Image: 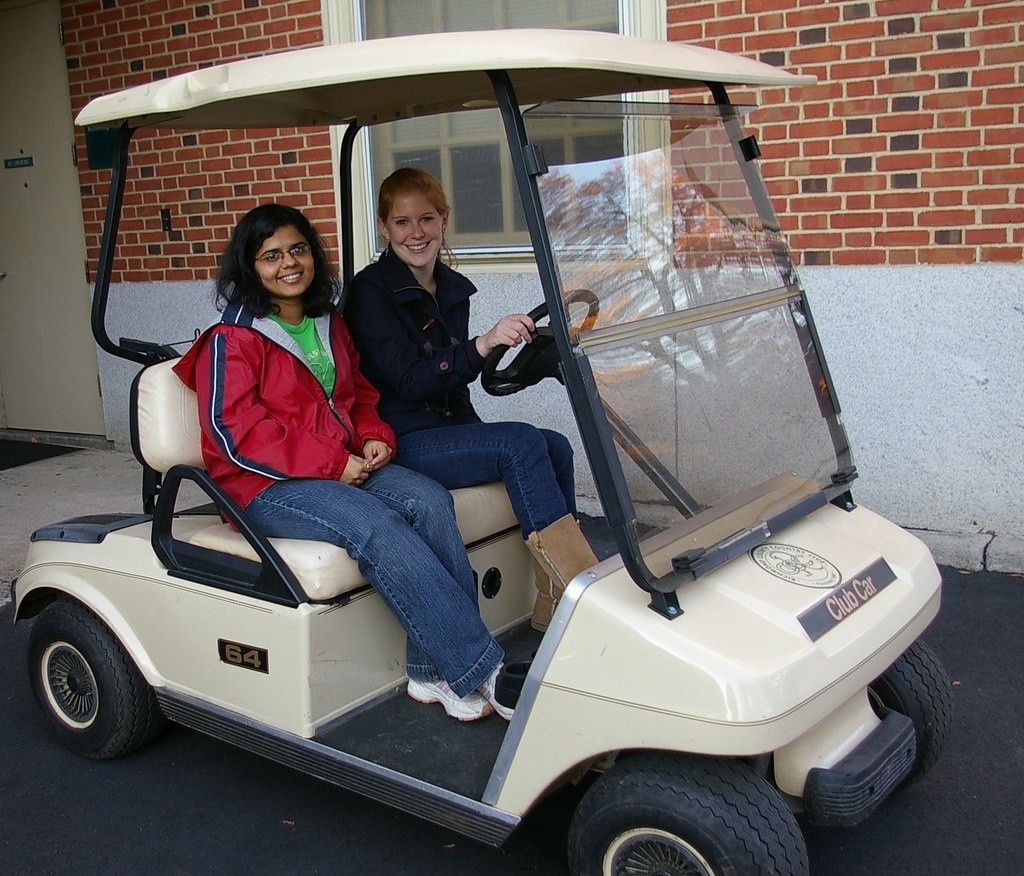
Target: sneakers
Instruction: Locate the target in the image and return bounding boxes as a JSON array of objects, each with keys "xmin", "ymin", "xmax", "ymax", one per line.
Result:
[
  {"xmin": 477, "ymin": 662, "xmax": 515, "ymax": 720},
  {"xmin": 407, "ymin": 678, "xmax": 494, "ymax": 721}
]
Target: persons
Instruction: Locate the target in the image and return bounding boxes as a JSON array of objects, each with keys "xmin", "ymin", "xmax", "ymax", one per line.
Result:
[
  {"xmin": 337, "ymin": 168, "xmax": 601, "ymax": 633},
  {"xmin": 172, "ymin": 204, "xmax": 524, "ymax": 722}
]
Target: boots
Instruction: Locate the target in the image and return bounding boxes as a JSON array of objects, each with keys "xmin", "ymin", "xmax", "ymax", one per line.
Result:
[
  {"xmin": 524, "ymin": 513, "xmax": 599, "ymax": 590},
  {"xmin": 530, "ymin": 553, "xmax": 563, "ymax": 632}
]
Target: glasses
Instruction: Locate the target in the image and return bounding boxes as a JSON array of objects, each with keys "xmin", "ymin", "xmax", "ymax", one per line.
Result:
[{"xmin": 254, "ymin": 244, "xmax": 312, "ymax": 265}]
